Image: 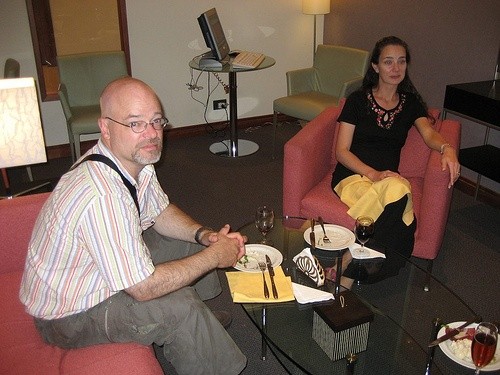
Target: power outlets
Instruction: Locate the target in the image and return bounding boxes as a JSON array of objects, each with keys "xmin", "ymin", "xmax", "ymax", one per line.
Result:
[{"xmin": 213, "ymin": 99, "xmax": 226, "ymax": 109}]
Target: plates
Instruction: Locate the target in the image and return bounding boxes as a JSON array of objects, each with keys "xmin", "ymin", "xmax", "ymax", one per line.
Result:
[
  {"xmin": 303, "ymin": 224, "xmax": 356, "ymax": 251},
  {"xmin": 233, "ymin": 244, "xmax": 283, "ymax": 272},
  {"xmin": 437, "ymin": 321, "xmax": 500, "ymax": 371}
]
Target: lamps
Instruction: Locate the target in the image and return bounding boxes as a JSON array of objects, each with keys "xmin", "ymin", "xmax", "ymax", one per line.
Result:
[
  {"xmin": 303, "ymin": 0, "xmax": 330, "ymax": 55},
  {"xmin": 0, "ymin": 78, "xmax": 49, "ymax": 182}
]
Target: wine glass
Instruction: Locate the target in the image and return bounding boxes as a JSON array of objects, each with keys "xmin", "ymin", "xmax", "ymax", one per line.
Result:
[
  {"xmin": 353, "ymin": 216, "xmax": 374, "ymax": 257},
  {"xmin": 471, "ymin": 322, "xmax": 498, "ymax": 375},
  {"xmin": 255, "ymin": 206, "xmax": 274, "ymax": 245}
]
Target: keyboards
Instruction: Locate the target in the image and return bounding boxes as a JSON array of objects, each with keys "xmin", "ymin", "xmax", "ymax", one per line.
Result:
[{"xmin": 233, "ymin": 52, "xmax": 266, "ymax": 69}]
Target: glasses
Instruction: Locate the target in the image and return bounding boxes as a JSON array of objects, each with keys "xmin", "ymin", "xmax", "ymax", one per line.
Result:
[{"xmin": 103, "ymin": 116, "xmax": 168, "ymax": 133}]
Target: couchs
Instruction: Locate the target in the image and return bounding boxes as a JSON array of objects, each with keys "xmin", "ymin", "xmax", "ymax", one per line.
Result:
[{"xmin": 0, "ymin": 193, "xmax": 164, "ymax": 375}]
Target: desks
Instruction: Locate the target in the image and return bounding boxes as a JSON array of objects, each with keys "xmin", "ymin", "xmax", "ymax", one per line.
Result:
[{"xmin": 190, "ymin": 51, "xmax": 276, "ymax": 157}]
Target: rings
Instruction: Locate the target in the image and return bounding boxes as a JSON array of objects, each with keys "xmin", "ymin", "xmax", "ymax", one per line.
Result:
[{"xmin": 457, "ymin": 172, "xmax": 461, "ymax": 173}]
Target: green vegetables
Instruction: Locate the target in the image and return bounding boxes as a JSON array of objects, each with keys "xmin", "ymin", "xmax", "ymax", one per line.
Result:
[{"xmin": 239, "ymin": 255, "xmax": 248, "ymax": 268}]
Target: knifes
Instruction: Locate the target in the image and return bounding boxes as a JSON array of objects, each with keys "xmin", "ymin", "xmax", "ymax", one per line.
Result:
[
  {"xmin": 309, "ymin": 217, "xmax": 315, "ymax": 250},
  {"xmin": 265, "ymin": 254, "xmax": 278, "ymax": 299},
  {"xmin": 426, "ymin": 319, "xmax": 478, "ymax": 347}
]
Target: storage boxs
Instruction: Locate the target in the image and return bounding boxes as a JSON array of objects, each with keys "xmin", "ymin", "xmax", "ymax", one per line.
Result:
[{"xmin": 311, "ymin": 290, "xmax": 374, "ymax": 362}]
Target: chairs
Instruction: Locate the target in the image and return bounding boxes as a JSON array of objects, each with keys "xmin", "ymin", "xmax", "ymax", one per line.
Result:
[
  {"xmin": 283, "ymin": 98, "xmax": 461, "ymax": 292},
  {"xmin": 273, "ymin": 45, "xmax": 370, "ymax": 160},
  {"xmin": 55, "ymin": 51, "xmax": 130, "ymax": 164}
]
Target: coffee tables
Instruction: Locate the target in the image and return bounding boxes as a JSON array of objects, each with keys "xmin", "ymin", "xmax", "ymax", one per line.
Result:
[{"xmin": 226, "ymin": 216, "xmax": 500, "ymax": 375}]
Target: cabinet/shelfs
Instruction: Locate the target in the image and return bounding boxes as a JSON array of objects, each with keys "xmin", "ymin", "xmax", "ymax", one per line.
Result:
[{"xmin": 443, "ymin": 78, "xmax": 500, "ymax": 200}]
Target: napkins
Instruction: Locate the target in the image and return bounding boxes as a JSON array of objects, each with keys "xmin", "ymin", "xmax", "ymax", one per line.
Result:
[
  {"xmin": 334, "ymin": 173, "xmax": 414, "ymax": 226},
  {"xmin": 349, "ymin": 243, "xmax": 386, "ymax": 260},
  {"xmin": 224, "ymin": 266, "xmax": 295, "ymax": 303},
  {"xmin": 292, "ymin": 282, "xmax": 335, "ymax": 305}
]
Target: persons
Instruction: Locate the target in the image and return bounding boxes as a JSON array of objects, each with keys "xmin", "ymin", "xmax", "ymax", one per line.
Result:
[
  {"xmin": 323, "ymin": 37, "xmax": 461, "ymax": 293},
  {"xmin": 18, "ymin": 77, "xmax": 249, "ymax": 374}
]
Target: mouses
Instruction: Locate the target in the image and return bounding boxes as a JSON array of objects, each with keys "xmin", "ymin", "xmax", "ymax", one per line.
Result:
[{"xmin": 228, "ymin": 49, "xmax": 241, "ymax": 55}]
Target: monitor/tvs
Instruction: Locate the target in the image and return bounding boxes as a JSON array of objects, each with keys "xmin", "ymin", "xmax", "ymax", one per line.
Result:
[{"xmin": 197, "ymin": 7, "xmax": 231, "ymax": 68}]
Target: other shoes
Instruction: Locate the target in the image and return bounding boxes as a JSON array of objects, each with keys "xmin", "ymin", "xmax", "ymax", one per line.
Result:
[{"xmin": 212, "ymin": 311, "xmax": 231, "ymax": 327}]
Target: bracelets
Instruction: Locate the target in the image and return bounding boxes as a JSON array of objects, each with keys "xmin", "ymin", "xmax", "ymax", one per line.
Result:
[
  {"xmin": 195, "ymin": 226, "xmax": 213, "ymax": 245},
  {"xmin": 440, "ymin": 143, "xmax": 451, "ymax": 154}
]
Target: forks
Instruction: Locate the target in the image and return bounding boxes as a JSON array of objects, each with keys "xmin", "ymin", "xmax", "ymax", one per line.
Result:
[
  {"xmin": 318, "ymin": 216, "xmax": 331, "ymax": 243},
  {"xmin": 258, "ymin": 261, "xmax": 270, "ymax": 299}
]
